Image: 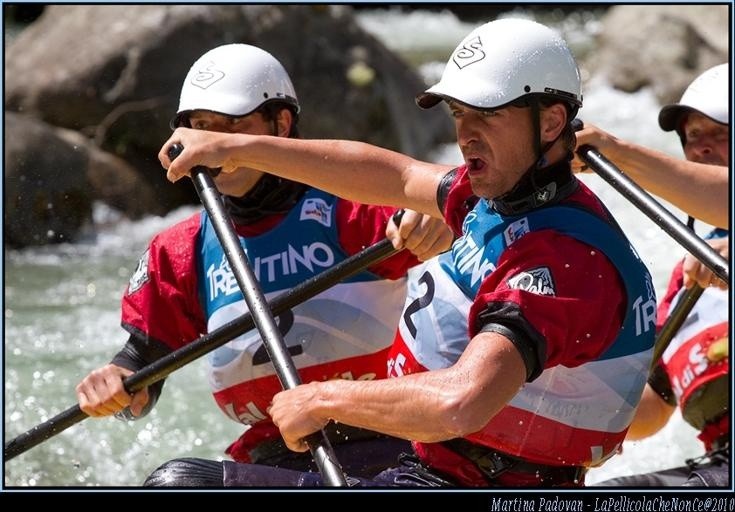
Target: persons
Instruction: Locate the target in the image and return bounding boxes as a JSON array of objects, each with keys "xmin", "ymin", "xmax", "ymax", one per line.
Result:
[
  {"xmin": 74, "ymin": 43, "xmax": 459, "ymax": 490},
  {"xmin": 592, "ymin": 60, "xmax": 728, "ymax": 487},
  {"xmin": 158, "ymin": 15, "xmax": 656, "ymax": 488},
  {"xmin": 568, "ymin": 117, "xmax": 729, "ymax": 230}
]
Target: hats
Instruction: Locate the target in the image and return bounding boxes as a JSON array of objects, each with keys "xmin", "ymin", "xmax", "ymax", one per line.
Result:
[
  {"xmin": 415, "ymin": 17, "xmax": 584, "ymax": 113},
  {"xmin": 174, "ymin": 42, "xmax": 303, "ymax": 118},
  {"xmin": 657, "ymin": 63, "xmax": 730, "ymax": 131}
]
